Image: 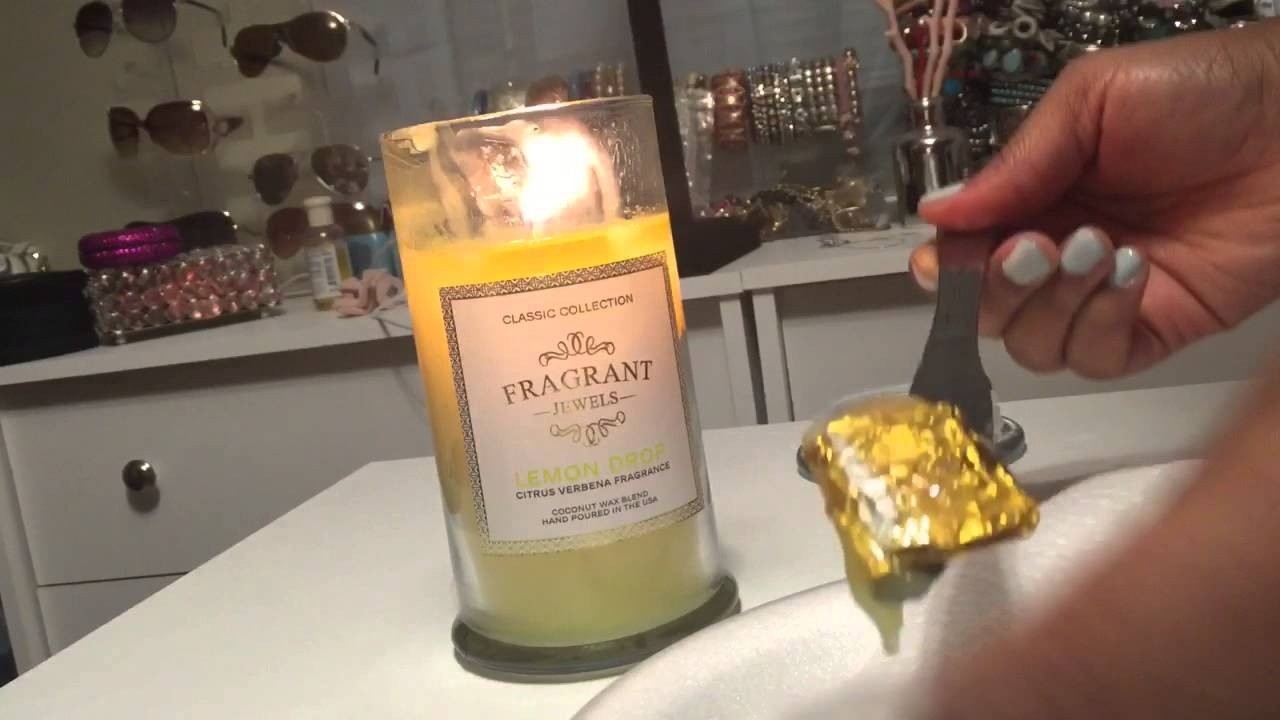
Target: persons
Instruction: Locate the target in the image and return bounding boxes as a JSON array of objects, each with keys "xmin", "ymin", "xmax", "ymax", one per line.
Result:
[{"xmin": 905, "ymin": 14, "xmax": 1280, "ymax": 720}]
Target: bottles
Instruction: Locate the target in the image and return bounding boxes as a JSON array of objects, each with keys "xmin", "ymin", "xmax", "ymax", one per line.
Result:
[{"xmin": 302, "ymin": 195, "xmax": 355, "ymax": 312}]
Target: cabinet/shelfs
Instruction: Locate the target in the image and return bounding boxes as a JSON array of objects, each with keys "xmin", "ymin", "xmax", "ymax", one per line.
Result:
[{"xmin": 0, "ymin": 213, "xmax": 1280, "ymax": 680}]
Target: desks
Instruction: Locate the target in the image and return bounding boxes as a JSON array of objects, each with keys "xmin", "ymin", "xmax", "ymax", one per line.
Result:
[{"xmin": 0, "ymin": 381, "xmax": 1250, "ymax": 720}]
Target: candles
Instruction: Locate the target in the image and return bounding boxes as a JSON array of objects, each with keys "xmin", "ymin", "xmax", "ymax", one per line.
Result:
[{"xmin": 403, "ymin": 131, "xmax": 724, "ymax": 646}]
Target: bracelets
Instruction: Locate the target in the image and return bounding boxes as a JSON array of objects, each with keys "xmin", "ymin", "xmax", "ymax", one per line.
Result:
[
  {"xmin": 672, "ymin": 48, "xmax": 862, "ymax": 159},
  {"xmin": 473, "ymin": 63, "xmax": 627, "ymax": 115}
]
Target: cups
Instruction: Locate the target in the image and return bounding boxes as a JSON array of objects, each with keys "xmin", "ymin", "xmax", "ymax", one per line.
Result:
[{"xmin": 379, "ymin": 93, "xmax": 744, "ymax": 684}]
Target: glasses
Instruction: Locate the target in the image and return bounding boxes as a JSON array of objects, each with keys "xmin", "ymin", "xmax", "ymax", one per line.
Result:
[
  {"xmin": 230, "ymin": 12, "xmax": 380, "ymax": 76},
  {"xmin": 71, "ymin": 0, "xmax": 228, "ymax": 59},
  {"xmin": 265, "ymin": 200, "xmax": 382, "ymax": 260},
  {"xmin": 109, "ymin": 101, "xmax": 241, "ymax": 156},
  {"xmin": 248, "ymin": 144, "xmax": 382, "ymax": 206}
]
